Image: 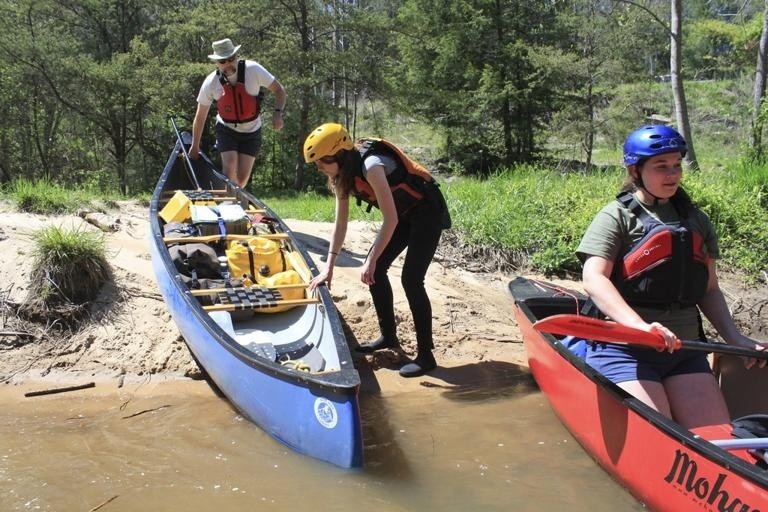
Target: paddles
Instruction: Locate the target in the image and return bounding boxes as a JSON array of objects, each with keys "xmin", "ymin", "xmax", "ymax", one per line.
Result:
[
  {"xmin": 166, "ymin": 114, "xmax": 217, "ymax": 206},
  {"xmin": 533, "ymin": 314, "xmax": 768, "ymax": 362}
]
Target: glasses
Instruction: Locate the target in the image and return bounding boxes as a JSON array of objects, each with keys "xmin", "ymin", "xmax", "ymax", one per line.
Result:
[{"xmin": 217, "ymin": 54, "xmax": 237, "ymax": 64}]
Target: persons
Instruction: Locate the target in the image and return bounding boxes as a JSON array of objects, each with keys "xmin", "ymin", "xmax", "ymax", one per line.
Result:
[
  {"xmin": 302, "ymin": 123, "xmax": 451, "ymax": 376},
  {"xmin": 187, "ymin": 38, "xmax": 286, "ymax": 186},
  {"xmin": 574, "ymin": 124, "xmax": 767, "ymax": 430}
]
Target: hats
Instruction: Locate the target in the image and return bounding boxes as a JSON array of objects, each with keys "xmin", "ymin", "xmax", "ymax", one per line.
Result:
[{"xmin": 208, "ymin": 38, "xmax": 241, "ymax": 60}]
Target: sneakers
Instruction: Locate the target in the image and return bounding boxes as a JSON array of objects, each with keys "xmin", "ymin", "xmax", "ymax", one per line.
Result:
[
  {"xmin": 355, "ymin": 334, "xmax": 399, "ymax": 353},
  {"xmin": 399, "ymin": 351, "xmax": 437, "ymax": 377}
]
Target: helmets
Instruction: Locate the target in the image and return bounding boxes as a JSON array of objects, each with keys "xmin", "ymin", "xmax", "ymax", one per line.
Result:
[
  {"xmin": 623, "ymin": 123, "xmax": 689, "ymax": 167},
  {"xmin": 302, "ymin": 123, "xmax": 354, "ymax": 164}
]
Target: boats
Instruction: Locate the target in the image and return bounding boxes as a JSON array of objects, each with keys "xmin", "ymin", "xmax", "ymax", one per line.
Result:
[
  {"xmin": 506, "ymin": 276, "xmax": 768, "ymax": 512},
  {"xmin": 149, "ymin": 130, "xmax": 365, "ymax": 470}
]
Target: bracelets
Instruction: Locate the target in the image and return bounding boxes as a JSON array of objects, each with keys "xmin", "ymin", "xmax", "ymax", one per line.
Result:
[
  {"xmin": 274, "ymin": 108, "xmax": 283, "ymax": 113},
  {"xmin": 328, "ymin": 251, "xmax": 338, "ymax": 255}
]
